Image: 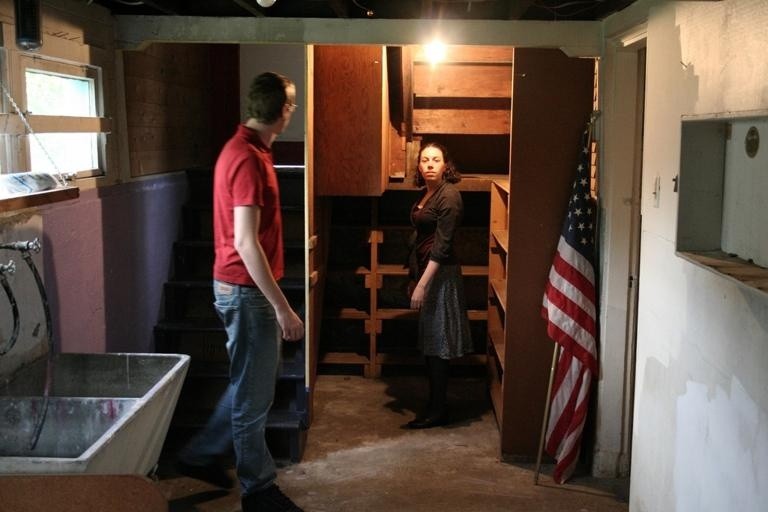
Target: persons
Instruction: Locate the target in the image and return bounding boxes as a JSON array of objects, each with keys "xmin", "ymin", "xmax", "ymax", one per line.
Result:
[
  {"xmin": 403, "ymin": 141, "xmax": 484, "ymax": 430},
  {"xmin": 175, "ymin": 70, "xmax": 307, "ymax": 512}
]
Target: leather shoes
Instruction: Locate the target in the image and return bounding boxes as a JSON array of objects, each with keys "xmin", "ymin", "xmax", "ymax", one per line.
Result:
[{"xmin": 407, "ymin": 403, "xmax": 452, "ymax": 428}]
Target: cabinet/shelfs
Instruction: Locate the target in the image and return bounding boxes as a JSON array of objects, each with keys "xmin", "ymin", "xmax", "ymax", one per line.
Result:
[
  {"xmin": 319, "ymin": 172, "xmax": 490, "ymax": 380},
  {"xmin": 487, "ymin": 173, "xmax": 581, "ymax": 462}
]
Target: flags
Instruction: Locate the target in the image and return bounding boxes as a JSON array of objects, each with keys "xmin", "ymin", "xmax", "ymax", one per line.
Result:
[{"xmin": 540, "ymin": 132, "xmax": 601, "ymax": 485}]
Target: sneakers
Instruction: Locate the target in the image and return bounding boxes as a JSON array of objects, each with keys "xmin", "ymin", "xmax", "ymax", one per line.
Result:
[
  {"xmin": 240, "ymin": 483, "xmax": 303, "ymax": 512},
  {"xmin": 164, "ymin": 441, "xmax": 235, "ymax": 489}
]
